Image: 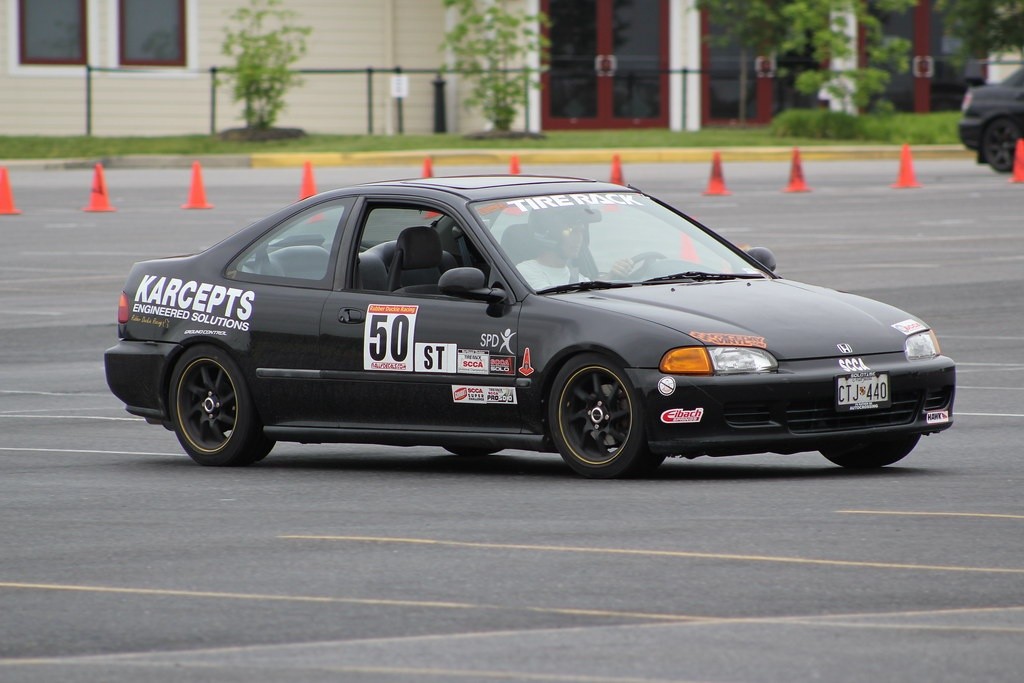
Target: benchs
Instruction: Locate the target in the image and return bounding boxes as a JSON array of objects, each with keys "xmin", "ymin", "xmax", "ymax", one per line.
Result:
[{"xmin": 256, "ymin": 239, "xmax": 454, "ymax": 287}]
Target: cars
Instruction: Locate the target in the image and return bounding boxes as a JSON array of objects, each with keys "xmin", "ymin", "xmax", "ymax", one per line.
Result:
[{"xmin": 959, "ymin": 67, "xmax": 1024, "ymax": 172}]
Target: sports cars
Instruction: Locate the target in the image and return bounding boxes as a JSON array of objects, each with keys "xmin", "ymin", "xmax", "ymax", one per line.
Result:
[{"xmin": 103, "ymin": 175, "xmax": 956, "ymax": 480}]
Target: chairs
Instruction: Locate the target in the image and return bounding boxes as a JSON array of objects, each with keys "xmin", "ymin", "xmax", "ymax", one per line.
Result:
[
  {"xmin": 501, "ymin": 225, "xmax": 539, "ymax": 265},
  {"xmin": 388, "ymin": 227, "xmax": 459, "ymax": 294}
]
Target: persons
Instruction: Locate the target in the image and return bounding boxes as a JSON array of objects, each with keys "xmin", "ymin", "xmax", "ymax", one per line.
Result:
[{"xmin": 513, "ymin": 207, "xmax": 638, "ymax": 295}]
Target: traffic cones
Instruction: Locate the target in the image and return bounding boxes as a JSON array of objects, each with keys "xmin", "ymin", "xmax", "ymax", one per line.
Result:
[
  {"xmin": 82, "ymin": 163, "xmax": 117, "ymax": 212},
  {"xmin": 890, "ymin": 143, "xmax": 924, "ymax": 189},
  {"xmin": 781, "ymin": 146, "xmax": 814, "ymax": 193},
  {"xmin": 700, "ymin": 150, "xmax": 731, "ymax": 196},
  {"xmin": 0, "ymin": 165, "xmax": 24, "ymax": 216},
  {"xmin": 509, "ymin": 154, "xmax": 521, "ymax": 175},
  {"xmin": 603, "ymin": 154, "xmax": 625, "ymax": 212},
  {"xmin": 180, "ymin": 160, "xmax": 213, "ymax": 209},
  {"xmin": 296, "ymin": 161, "xmax": 318, "ymax": 201},
  {"xmin": 420, "ymin": 156, "xmax": 439, "ymax": 219},
  {"xmin": 1007, "ymin": 137, "xmax": 1024, "ymax": 182}
]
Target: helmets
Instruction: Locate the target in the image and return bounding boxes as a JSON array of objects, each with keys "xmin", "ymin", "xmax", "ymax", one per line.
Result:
[{"xmin": 529, "ymin": 206, "xmax": 600, "ymax": 262}]
